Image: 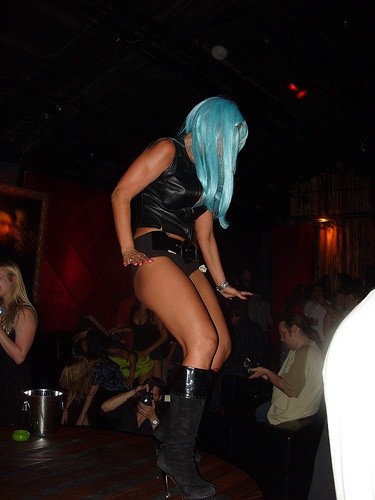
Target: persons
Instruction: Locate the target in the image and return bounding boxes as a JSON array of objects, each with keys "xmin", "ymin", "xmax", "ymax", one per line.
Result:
[
  {"xmin": 86, "ymin": 329, "xmax": 155, "ymax": 386},
  {"xmin": 219, "ymin": 270, "xmax": 363, "ymax": 500},
  {"xmin": 59, "ymin": 357, "xmax": 128, "ymax": 425},
  {"xmin": 111, "ymin": 96, "xmax": 255, "ymax": 500},
  {"xmin": 0, "ymin": 261, "xmax": 40, "ymax": 422},
  {"xmin": 100, "ymin": 376, "xmax": 170, "ymax": 437},
  {"xmin": 110, "ymin": 298, "xmax": 169, "ymax": 380}
]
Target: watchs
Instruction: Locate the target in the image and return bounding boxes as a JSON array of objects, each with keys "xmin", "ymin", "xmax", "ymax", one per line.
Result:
[
  {"xmin": 151, "ymin": 419, "xmax": 159, "ymax": 426},
  {"xmin": 216, "ymin": 280, "xmax": 230, "ymax": 291}
]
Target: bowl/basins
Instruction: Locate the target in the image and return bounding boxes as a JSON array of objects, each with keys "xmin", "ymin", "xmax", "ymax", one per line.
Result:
[{"xmin": 12, "ymin": 430, "xmax": 31, "ymax": 442}]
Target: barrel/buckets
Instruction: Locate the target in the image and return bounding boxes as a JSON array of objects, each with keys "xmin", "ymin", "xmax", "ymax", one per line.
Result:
[{"xmin": 23, "ymin": 389, "xmax": 64, "ymax": 434}]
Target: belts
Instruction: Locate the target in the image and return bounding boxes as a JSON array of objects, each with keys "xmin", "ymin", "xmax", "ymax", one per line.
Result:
[{"xmin": 152, "ymin": 231, "xmax": 202, "ymax": 263}]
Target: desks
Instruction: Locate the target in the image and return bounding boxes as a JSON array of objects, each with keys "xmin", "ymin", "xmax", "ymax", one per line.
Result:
[{"xmin": 0, "ymin": 423, "xmax": 264, "ymax": 500}]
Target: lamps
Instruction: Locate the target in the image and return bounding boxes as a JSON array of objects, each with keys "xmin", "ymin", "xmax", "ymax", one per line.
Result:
[{"xmin": 210, "ymin": 39, "xmax": 228, "ymax": 63}]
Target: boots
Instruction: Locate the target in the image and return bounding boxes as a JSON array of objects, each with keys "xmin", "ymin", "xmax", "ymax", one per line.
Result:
[{"xmin": 151, "ymin": 365, "xmax": 216, "ymax": 499}]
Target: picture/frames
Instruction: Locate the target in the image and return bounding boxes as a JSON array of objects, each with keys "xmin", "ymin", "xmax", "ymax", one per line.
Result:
[{"xmin": 0, "ymin": 182, "xmax": 48, "ymax": 306}]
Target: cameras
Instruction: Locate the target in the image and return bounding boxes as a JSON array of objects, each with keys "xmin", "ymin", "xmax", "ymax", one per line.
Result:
[
  {"xmin": 142, "ymin": 389, "xmax": 153, "ymax": 406},
  {"xmin": 244, "ymin": 357, "xmax": 260, "ymax": 375}
]
